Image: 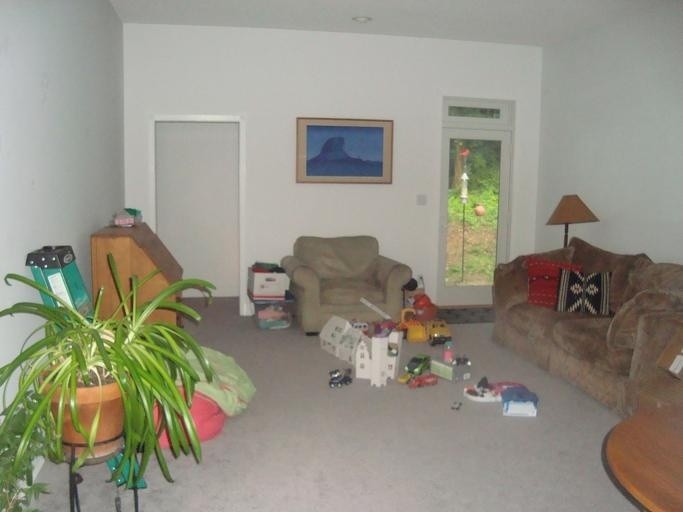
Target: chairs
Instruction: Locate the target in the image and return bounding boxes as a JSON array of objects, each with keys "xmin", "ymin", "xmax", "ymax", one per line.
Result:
[{"xmin": 281, "ymin": 235, "xmax": 411, "ymax": 336}]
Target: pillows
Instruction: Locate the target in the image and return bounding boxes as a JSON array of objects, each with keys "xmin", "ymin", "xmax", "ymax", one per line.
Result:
[
  {"xmin": 605, "ymin": 288, "xmax": 683, "ymax": 349},
  {"xmin": 555, "ymin": 266, "xmax": 612, "ymax": 317},
  {"xmin": 523, "ymin": 255, "xmax": 581, "ymax": 310}
]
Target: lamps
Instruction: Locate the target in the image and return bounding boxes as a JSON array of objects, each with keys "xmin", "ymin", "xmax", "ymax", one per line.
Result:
[{"xmin": 546, "ymin": 194, "xmax": 599, "ymax": 249}]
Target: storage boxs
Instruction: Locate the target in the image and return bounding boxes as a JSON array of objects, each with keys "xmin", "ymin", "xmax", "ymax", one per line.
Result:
[
  {"xmin": 253, "ymin": 291, "xmax": 293, "ymax": 331},
  {"xmin": 247, "ymin": 268, "xmax": 289, "ymax": 300}
]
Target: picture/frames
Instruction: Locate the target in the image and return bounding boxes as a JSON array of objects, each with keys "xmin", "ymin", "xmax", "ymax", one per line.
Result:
[{"xmin": 296, "ymin": 118, "xmax": 394, "ymax": 185}]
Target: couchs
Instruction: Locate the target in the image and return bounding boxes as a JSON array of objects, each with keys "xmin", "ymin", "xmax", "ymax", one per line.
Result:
[{"xmin": 492, "ymin": 239, "xmax": 683, "ymax": 418}]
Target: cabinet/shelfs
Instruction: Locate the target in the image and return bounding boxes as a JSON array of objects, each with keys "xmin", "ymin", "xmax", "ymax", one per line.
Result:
[{"xmin": 90, "ymin": 223, "xmax": 184, "ymax": 331}]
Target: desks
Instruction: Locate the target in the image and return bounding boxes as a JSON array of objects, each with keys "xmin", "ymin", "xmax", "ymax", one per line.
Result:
[
  {"xmin": 56, "ymin": 438, "xmax": 139, "ymax": 512},
  {"xmin": 602, "ymin": 406, "xmax": 683, "ymax": 512}
]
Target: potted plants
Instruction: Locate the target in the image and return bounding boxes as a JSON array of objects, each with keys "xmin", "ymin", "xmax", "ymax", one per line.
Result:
[{"xmin": 0, "ymin": 253, "xmax": 216, "ymax": 512}]
